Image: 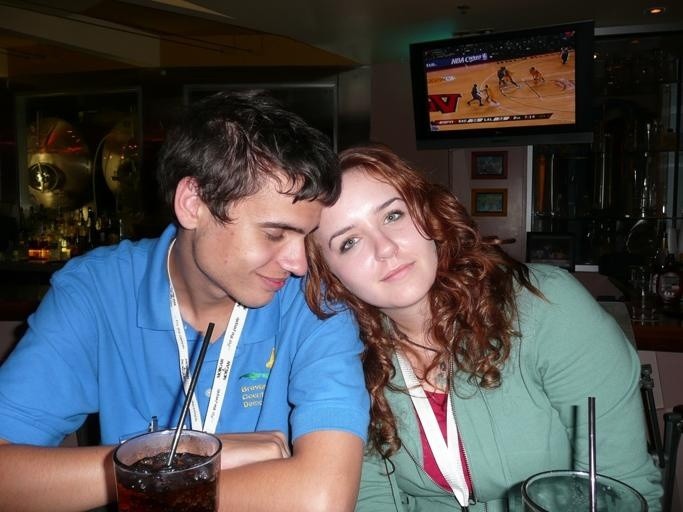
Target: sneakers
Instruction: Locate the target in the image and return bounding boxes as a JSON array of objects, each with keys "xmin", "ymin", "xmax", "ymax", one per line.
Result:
[
  {"xmin": 499, "ymin": 80, "xmax": 547, "ymax": 90},
  {"xmin": 466, "ymin": 101, "xmax": 500, "ymax": 107}
]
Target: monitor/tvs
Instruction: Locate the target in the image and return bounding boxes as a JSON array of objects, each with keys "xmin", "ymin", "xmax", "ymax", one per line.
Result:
[{"xmin": 409, "ymin": 19, "xmax": 595, "ymax": 151}]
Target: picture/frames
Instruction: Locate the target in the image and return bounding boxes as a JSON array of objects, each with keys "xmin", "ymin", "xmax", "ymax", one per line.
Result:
[
  {"xmin": 472, "ymin": 188, "xmax": 508, "ymax": 217},
  {"xmin": 472, "ymin": 151, "xmax": 507, "ymax": 179}
]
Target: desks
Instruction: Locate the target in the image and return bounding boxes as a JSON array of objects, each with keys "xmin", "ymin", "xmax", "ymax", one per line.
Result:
[{"xmin": 572, "ymin": 272, "xmax": 624, "ymax": 302}]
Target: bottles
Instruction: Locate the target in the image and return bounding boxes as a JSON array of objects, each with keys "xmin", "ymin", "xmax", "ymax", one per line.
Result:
[
  {"xmin": 12, "ymin": 204, "xmax": 117, "ymax": 261},
  {"xmin": 648, "ymin": 225, "xmax": 682, "ymax": 312}
]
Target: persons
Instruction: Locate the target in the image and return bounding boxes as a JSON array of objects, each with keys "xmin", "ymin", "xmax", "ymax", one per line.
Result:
[
  {"xmin": 0, "ymin": 86, "xmax": 376, "ymax": 512},
  {"xmin": 502, "ymin": 65, "xmax": 518, "ymax": 87},
  {"xmin": 528, "ymin": 67, "xmax": 544, "ymax": 84},
  {"xmin": 558, "ymin": 45, "xmax": 569, "ymax": 65},
  {"xmin": 478, "ymin": 84, "xmax": 497, "ymax": 105},
  {"xmin": 496, "ymin": 67, "xmax": 507, "ymax": 90},
  {"xmin": 466, "ymin": 84, "xmax": 484, "ymax": 107},
  {"xmin": 303, "ymin": 143, "xmax": 667, "ymax": 512}
]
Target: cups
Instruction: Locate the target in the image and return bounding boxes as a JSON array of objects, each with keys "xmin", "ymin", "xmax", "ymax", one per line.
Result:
[
  {"xmin": 626, "ymin": 264, "xmax": 661, "ymax": 326},
  {"xmin": 521, "ymin": 469, "xmax": 648, "ymax": 512},
  {"xmin": 114, "ymin": 429, "xmax": 222, "ymax": 512}
]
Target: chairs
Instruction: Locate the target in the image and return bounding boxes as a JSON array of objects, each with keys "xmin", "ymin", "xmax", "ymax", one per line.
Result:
[{"xmin": 637, "ymin": 351, "xmax": 683, "ymax": 512}]
